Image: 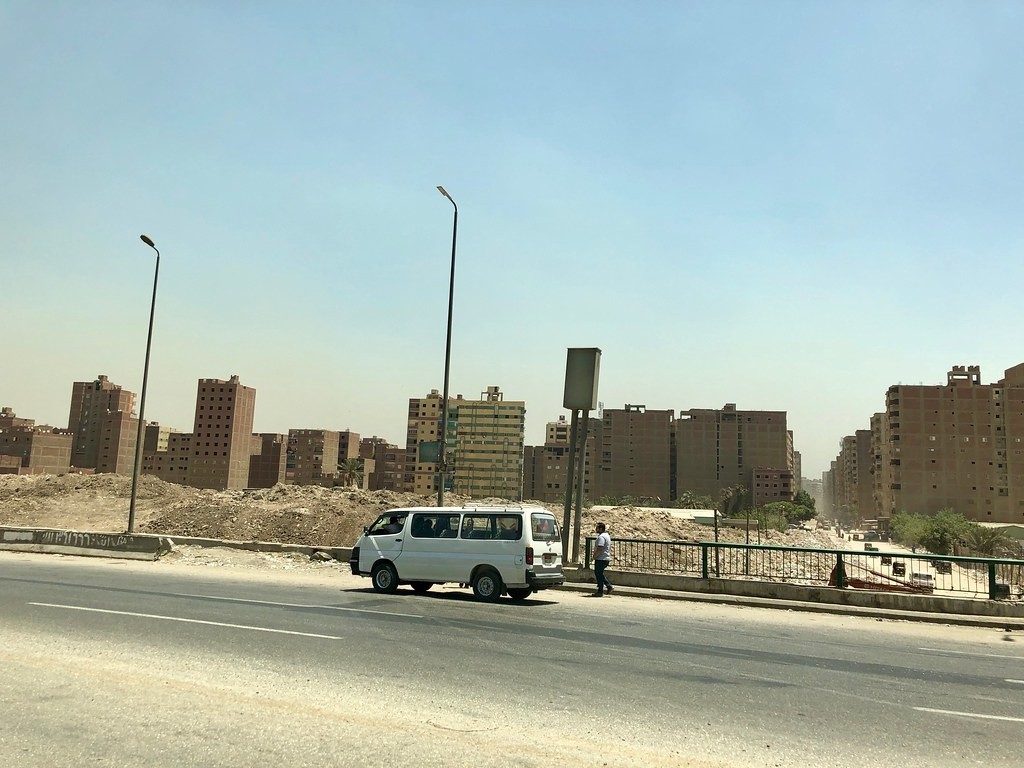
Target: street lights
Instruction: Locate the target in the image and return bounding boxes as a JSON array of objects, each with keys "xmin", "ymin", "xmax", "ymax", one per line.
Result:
[
  {"xmin": 437, "ymin": 184, "xmax": 459, "ymax": 507},
  {"xmin": 128, "ymin": 234, "xmax": 160, "ymax": 531}
]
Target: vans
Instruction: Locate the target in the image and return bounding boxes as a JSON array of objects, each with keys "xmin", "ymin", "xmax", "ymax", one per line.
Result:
[{"xmin": 350, "ymin": 502, "xmax": 568, "ymax": 604}]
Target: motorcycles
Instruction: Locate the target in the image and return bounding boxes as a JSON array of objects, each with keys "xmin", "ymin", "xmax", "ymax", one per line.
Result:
[
  {"xmin": 869, "ymin": 547, "xmax": 879, "ymax": 558},
  {"xmin": 853, "ymin": 535, "xmax": 859, "ymax": 541},
  {"xmin": 930, "ymin": 559, "xmax": 942, "ymax": 568},
  {"xmin": 864, "ymin": 543, "xmax": 872, "ymax": 551},
  {"xmin": 937, "ymin": 562, "xmax": 952, "ymax": 575},
  {"xmin": 880, "ymin": 553, "xmax": 892, "ymax": 567},
  {"xmin": 892, "ymin": 562, "xmax": 905, "ymax": 577}
]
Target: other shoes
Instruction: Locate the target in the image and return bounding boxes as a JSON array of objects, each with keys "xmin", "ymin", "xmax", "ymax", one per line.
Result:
[
  {"xmin": 606, "ymin": 586, "xmax": 614, "ymax": 594},
  {"xmin": 592, "ymin": 592, "xmax": 604, "ymax": 597}
]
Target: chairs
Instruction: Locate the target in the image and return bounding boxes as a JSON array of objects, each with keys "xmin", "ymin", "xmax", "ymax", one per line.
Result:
[{"xmin": 415, "ymin": 518, "xmax": 519, "ymax": 539}]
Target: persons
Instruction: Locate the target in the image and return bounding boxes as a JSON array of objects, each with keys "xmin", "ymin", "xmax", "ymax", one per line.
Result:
[
  {"xmin": 459, "ymin": 519, "xmax": 476, "ymax": 588},
  {"xmin": 537, "ymin": 519, "xmax": 549, "ymax": 533},
  {"xmin": 591, "ymin": 522, "xmax": 614, "ymax": 596},
  {"xmin": 382, "ymin": 516, "xmax": 403, "ymax": 534}
]
